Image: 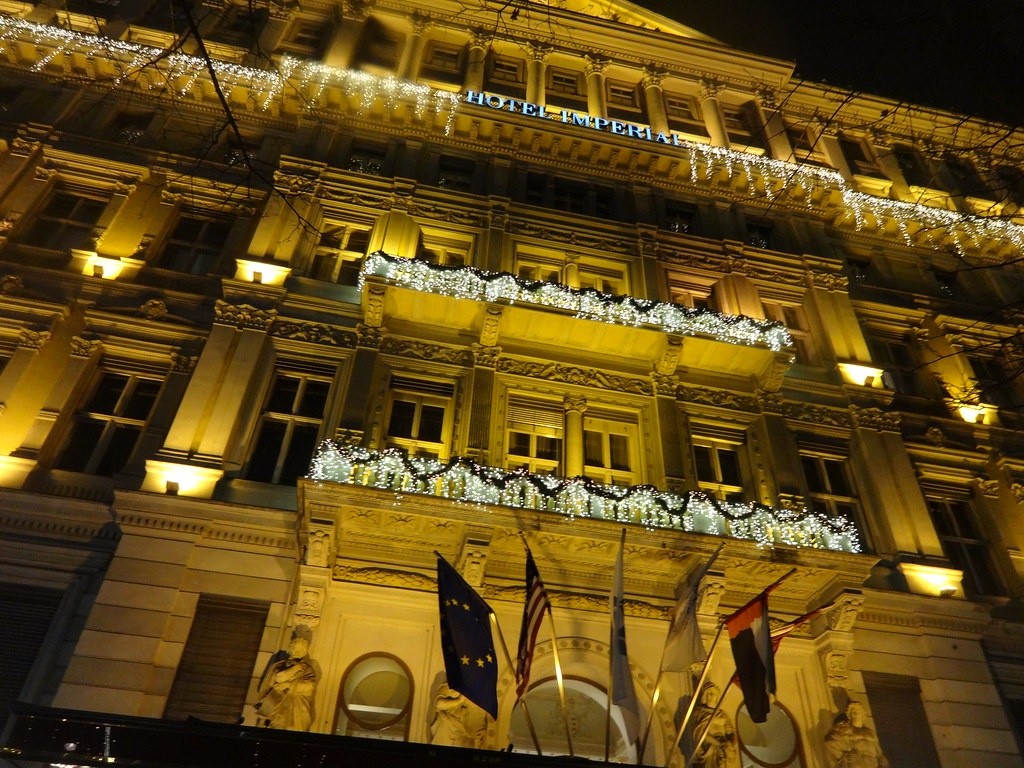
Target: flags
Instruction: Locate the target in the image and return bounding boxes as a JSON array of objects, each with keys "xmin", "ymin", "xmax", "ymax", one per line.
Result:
[
  {"xmin": 731, "ymin": 602, "xmax": 834, "ymax": 697},
  {"xmin": 610, "ymin": 546, "xmax": 649, "ymax": 748},
  {"xmin": 437, "ymin": 556, "xmax": 499, "ymax": 721},
  {"xmin": 726, "ymin": 594, "xmax": 777, "ymax": 724},
  {"xmin": 515, "ymin": 547, "xmax": 551, "ymax": 698},
  {"xmin": 660, "ymin": 564, "xmax": 709, "ymax": 673}
]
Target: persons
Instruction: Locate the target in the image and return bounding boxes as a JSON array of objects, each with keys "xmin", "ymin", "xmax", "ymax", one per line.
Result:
[
  {"xmin": 254, "ymin": 636, "xmax": 317, "ymax": 732},
  {"xmin": 687, "ymin": 685, "xmax": 742, "ymax": 768},
  {"xmin": 822, "ymin": 700, "xmax": 889, "ymax": 768}
]
